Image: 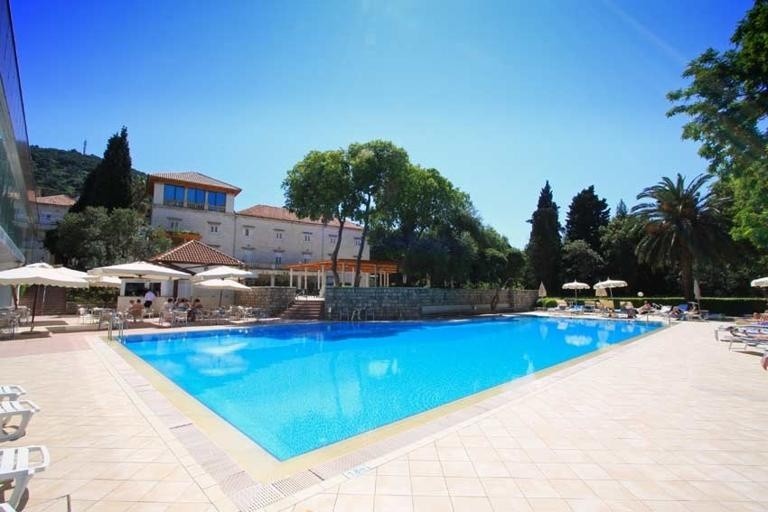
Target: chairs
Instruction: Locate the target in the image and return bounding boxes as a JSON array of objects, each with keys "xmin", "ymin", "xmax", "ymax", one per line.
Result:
[
  {"xmin": 76, "ymin": 303, "xmax": 263, "ymax": 326},
  {"xmin": 340, "ymin": 298, "xmax": 376, "ymax": 320},
  {"xmin": 0, "ymin": 384, "xmax": 49, "ymax": 512},
  {"xmin": 716, "ymin": 313, "xmax": 768, "ymax": 352},
  {"xmin": 549, "ymin": 299, "xmax": 707, "ymax": 322},
  {"xmin": 0, "ymin": 306, "xmax": 30, "ymax": 339}
]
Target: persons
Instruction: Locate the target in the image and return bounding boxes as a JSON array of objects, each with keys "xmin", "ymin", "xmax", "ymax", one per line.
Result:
[
  {"xmin": 124, "ymin": 289, "xmax": 204, "ymax": 323},
  {"xmin": 635, "ymin": 301, "xmax": 652, "ymax": 315},
  {"xmin": 729, "ymin": 328, "xmax": 767, "ymax": 340},
  {"xmin": 671, "ymin": 306, "xmax": 698, "ymax": 315},
  {"xmin": 751, "ymin": 310, "xmax": 767, "ymax": 322}
]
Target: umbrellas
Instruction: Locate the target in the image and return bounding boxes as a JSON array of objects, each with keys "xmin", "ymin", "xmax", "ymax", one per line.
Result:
[
  {"xmin": 0, "ymin": 261, "xmax": 89, "ymax": 333},
  {"xmin": 86, "ymin": 258, "xmax": 194, "ymax": 282},
  {"xmin": 193, "ymin": 279, "xmax": 251, "ymax": 308},
  {"xmin": 189, "ymin": 264, "xmax": 253, "ymax": 280},
  {"xmin": 560, "ymin": 279, "xmax": 591, "ymax": 303},
  {"xmin": 594, "ymin": 278, "xmax": 607, "ymax": 301},
  {"xmin": 536, "ymin": 280, "xmax": 548, "ymax": 312},
  {"xmin": 692, "ymin": 279, "xmax": 702, "ymax": 311},
  {"xmin": 748, "ymin": 276, "xmax": 767, "ymax": 289},
  {"xmin": 53, "ymin": 264, "xmax": 123, "ymax": 290},
  {"xmin": 592, "ymin": 276, "xmax": 629, "ymax": 297}
]
